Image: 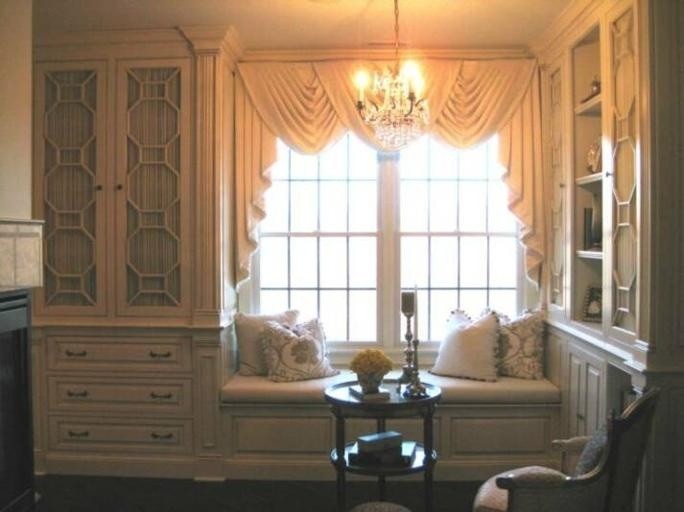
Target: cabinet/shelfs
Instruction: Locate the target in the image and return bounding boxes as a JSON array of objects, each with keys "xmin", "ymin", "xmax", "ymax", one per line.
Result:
[
  {"xmin": 567, "ymin": 18, "xmax": 603, "ymax": 333},
  {"xmin": 597, "ymin": 1, "xmax": 645, "ymax": 348},
  {"xmin": 24, "ymin": 330, "xmax": 198, "ymax": 480},
  {"xmin": 28, "ymin": 60, "xmax": 195, "ymax": 329},
  {"xmin": 565, "ymin": 342, "xmax": 607, "ymax": 440}
]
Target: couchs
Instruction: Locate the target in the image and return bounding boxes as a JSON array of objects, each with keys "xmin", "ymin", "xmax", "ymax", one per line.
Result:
[{"xmin": 472, "ymin": 382, "xmax": 666, "ymax": 512}]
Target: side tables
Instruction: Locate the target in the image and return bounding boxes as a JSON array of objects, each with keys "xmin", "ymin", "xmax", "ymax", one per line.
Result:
[{"xmin": 322, "ymin": 372, "xmax": 443, "ymax": 511}]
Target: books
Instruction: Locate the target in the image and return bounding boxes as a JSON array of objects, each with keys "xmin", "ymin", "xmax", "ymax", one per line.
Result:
[
  {"xmin": 348, "ymin": 441, "xmax": 417, "ymax": 466},
  {"xmin": 584, "ymin": 208, "xmax": 592, "ymax": 250},
  {"xmin": 349, "ymin": 385, "xmax": 390, "ymax": 401}
]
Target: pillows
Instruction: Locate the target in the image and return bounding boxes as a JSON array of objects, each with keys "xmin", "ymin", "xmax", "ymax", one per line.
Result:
[
  {"xmin": 231, "ymin": 309, "xmax": 301, "ymax": 378},
  {"xmin": 427, "ymin": 307, "xmax": 501, "ymax": 384},
  {"xmin": 259, "ymin": 314, "xmax": 341, "ymax": 382},
  {"xmin": 480, "ymin": 308, "xmax": 548, "ymax": 382}
]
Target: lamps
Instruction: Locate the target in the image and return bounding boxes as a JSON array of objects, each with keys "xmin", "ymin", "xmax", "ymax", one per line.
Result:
[{"xmin": 351, "ymin": 1, "xmax": 432, "ymax": 151}]
